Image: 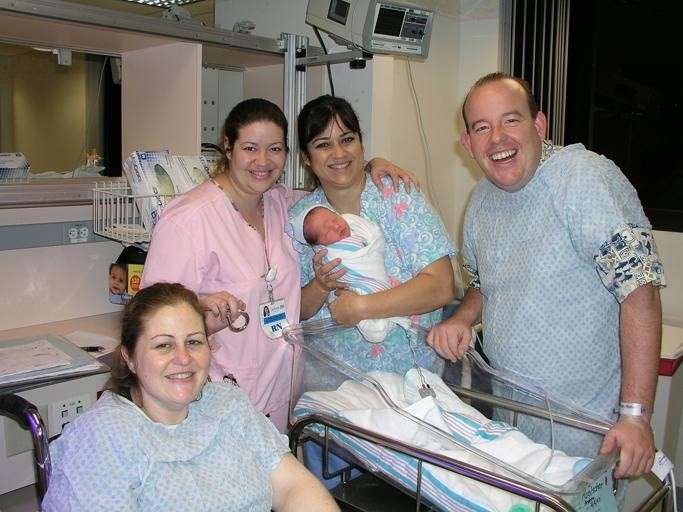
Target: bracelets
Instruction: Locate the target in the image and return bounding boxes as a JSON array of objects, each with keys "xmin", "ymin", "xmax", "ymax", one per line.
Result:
[{"xmin": 614, "ymin": 403, "xmax": 652, "ymax": 418}]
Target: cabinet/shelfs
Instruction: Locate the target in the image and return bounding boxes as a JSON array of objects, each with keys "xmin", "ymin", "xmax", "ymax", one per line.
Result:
[{"xmin": 0, "ymin": 1, "xmax": 327, "ymax": 252}]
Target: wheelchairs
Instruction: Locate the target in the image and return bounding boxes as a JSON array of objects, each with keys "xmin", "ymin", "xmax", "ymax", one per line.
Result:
[{"xmin": 0, "ymin": 375, "xmax": 212, "ymax": 512}]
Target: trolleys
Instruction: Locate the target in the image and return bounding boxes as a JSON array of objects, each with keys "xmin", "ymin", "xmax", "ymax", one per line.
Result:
[{"xmin": 288, "ymin": 380, "xmax": 677, "ymax": 512}]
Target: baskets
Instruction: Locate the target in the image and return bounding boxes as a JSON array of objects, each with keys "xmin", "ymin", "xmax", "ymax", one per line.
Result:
[{"xmin": 92, "ymin": 181, "xmax": 185, "ymax": 244}]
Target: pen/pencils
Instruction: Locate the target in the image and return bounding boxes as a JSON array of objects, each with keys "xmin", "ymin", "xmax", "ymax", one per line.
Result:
[{"xmin": 81, "ymin": 346, "xmax": 104, "ymax": 352}]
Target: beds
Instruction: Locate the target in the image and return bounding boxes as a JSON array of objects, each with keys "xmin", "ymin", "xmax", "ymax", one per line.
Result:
[{"xmin": 278, "ymin": 313, "xmax": 677, "ymax": 512}]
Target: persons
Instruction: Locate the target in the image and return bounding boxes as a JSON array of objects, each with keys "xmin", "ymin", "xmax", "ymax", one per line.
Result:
[
  {"xmin": 303, "ymin": 206, "xmax": 411, "ymax": 343},
  {"xmin": 138, "ymin": 99, "xmax": 419, "ymax": 442},
  {"xmin": 284, "ymin": 95, "xmax": 459, "ymax": 491},
  {"xmin": 425, "ymin": 73, "xmax": 662, "ymax": 479},
  {"xmin": 41, "ymin": 284, "xmax": 341, "ymax": 512}
]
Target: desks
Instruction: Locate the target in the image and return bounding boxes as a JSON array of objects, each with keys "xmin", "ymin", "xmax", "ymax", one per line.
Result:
[{"xmin": 0, "ymin": 309, "xmax": 129, "ymax": 498}]
